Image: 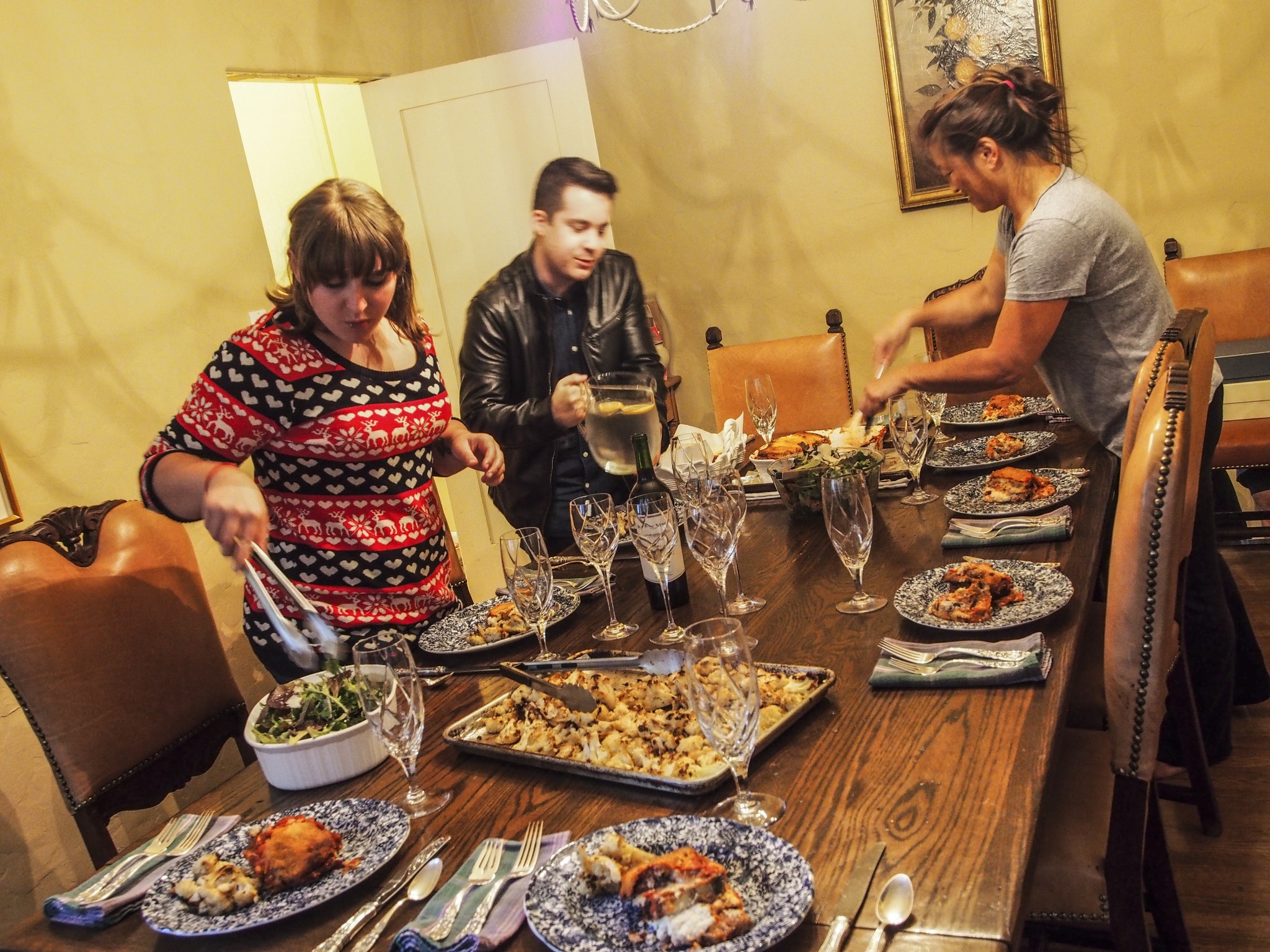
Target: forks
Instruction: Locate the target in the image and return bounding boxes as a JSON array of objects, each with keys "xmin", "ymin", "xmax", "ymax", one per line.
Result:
[
  {"xmin": 75, "ymin": 805, "xmax": 215, "ymax": 905},
  {"xmin": 552, "ymin": 574, "xmax": 600, "ymax": 589},
  {"xmin": 888, "ymin": 654, "xmax": 1022, "ymax": 676},
  {"xmin": 949, "ymin": 516, "xmax": 1068, "ymax": 539},
  {"xmin": 875, "ymin": 637, "xmax": 1031, "ymax": 661},
  {"xmin": 430, "ymin": 817, "xmax": 546, "ymax": 942}
]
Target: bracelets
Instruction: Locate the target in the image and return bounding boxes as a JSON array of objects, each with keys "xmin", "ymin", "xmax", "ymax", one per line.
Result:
[{"xmin": 203, "ymin": 462, "xmax": 239, "ymax": 493}]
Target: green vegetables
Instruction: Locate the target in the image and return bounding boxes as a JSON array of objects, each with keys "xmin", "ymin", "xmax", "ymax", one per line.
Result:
[
  {"xmin": 773, "ymin": 440, "xmax": 877, "ymax": 476},
  {"xmin": 251, "ymin": 657, "xmax": 390, "ymax": 744}
]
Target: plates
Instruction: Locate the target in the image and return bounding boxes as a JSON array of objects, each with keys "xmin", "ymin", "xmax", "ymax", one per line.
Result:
[
  {"xmin": 418, "ymin": 588, "xmax": 581, "ymax": 655},
  {"xmin": 893, "ymin": 559, "xmax": 1075, "ymax": 630},
  {"xmin": 940, "ymin": 396, "xmax": 1053, "ymax": 424},
  {"xmin": 926, "ymin": 430, "xmax": 1058, "ymax": 470},
  {"xmin": 582, "ymin": 499, "xmax": 705, "ymax": 546},
  {"xmin": 943, "ymin": 469, "xmax": 1081, "ymax": 516},
  {"xmin": 871, "ymin": 412, "xmax": 903, "ymax": 427},
  {"xmin": 524, "ymin": 815, "xmax": 817, "ymax": 951},
  {"xmin": 143, "ymin": 797, "xmax": 412, "ymax": 938}
]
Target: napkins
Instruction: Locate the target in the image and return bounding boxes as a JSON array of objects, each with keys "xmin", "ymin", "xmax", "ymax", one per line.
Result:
[
  {"xmin": 493, "ymin": 564, "xmax": 620, "ymax": 602},
  {"xmin": 867, "ymin": 632, "xmax": 1055, "ymax": 683},
  {"xmin": 41, "ymin": 810, "xmax": 241, "ymax": 928},
  {"xmin": 942, "ymin": 502, "xmax": 1073, "ymax": 547},
  {"xmin": 390, "ymin": 829, "xmax": 571, "ymax": 952}
]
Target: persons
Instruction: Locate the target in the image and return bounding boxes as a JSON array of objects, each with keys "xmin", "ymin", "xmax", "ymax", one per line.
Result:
[
  {"xmin": 857, "ymin": 61, "xmax": 1270, "ymax": 783},
  {"xmin": 137, "ymin": 176, "xmax": 506, "ymax": 687},
  {"xmin": 459, "ymin": 156, "xmax": 670, "ymax": 563}
]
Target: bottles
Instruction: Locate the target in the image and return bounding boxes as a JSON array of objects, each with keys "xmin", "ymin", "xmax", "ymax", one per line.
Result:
[{"xmin": 626, "ymin": 432, "xmax": 691, "ymax": 610}]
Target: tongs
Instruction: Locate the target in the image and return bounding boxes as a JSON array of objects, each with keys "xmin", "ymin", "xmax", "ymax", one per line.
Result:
[
  {"xmin": 499, "ymin": 647, "xmax": 685, "ymax": 712},
  {"xmin": 226, "ymin": 527, "xmax": 342, "ymax": 666}
]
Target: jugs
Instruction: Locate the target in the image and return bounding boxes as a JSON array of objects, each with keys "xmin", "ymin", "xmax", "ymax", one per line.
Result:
[{"xmin": 571, "ymin": 368, "xmax": 664, "ymax": 476}]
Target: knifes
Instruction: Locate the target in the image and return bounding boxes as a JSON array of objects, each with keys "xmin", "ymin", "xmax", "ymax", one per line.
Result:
[
  {"xmin": 310, "ymin": 835, "xmax": 451, "ymax": 952},
  {"xmin": 547, "ymin": 551, "xmax": 640, "ymax": 566},
  {"xmin": 393, "ymin": 663, "xmax": 500, "ymax": 676},
  {"xmin": 818, "ymin": 839, "xmax": 887, "ymax": 952}
]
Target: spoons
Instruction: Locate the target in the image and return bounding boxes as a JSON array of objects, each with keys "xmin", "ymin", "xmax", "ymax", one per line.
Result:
[
  {"xmin": 348, "ymin": 857, "xmax": 443, "ymax": 952},
  {"xmin": 398, "ymin": 672, "xmax": 454, "ymax": 686},
  {"xmin": 550, "ymin": 559, "xmax": 598, "ymax": 569},
  {"xmin": 553, "ymin": 580, "xmax": 597, "ymax": 592},
  {"xmin": 963, "ymin": 555, "xmax": 1062, "ymax": 568},
  {"xmin": 862, "ymin": 873, "xmax": 913, "ymax": 952}
]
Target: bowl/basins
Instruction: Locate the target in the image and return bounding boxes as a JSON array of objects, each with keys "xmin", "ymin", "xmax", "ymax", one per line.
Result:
[
  {"xmin": 242, "ymin": 660, "xmax": 397, "ymax": 794},
  {"xmin": 766, "ymin": 447, "xmax": 886, "ymax": 521}
]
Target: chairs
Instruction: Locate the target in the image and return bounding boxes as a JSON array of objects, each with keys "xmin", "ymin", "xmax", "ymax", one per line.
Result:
[
  {"xmin": 921, "ymin": 263, "xmax": 1067, "ymax": 402},
  {"xmin": 1162, "ymin": 235, "xmax": 1270, "ymax": 552},
  {"xmin": 0, "ymin": 493, "xmax": 262, "ymax": 868},
  {"xmin": 701, "ymin": 309, "xmax": 855, "ymax": 441}
]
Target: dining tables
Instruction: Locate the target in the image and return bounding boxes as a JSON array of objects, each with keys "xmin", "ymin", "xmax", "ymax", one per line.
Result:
[{"xmin": 0, "ymin": 409, "xmax": 1119, "ymax": 952}]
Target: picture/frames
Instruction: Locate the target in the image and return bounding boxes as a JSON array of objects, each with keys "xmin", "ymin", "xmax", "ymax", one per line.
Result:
[{"xmin": 874, "ymin": 0, "xmax": 1077, "ymax": 211}]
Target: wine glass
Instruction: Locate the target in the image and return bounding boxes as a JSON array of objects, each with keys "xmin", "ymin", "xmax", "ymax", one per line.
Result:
[
  {"xmin": 744, "ymin": 374, "xmax": 777, "ymax": 442},
  {"xmin": 667, "ymin": 431, "xmax": 712, "ymax": 531},
  {"xmin": 569, "ymin": 492, "xmax": 641, "ymax": 642},
  {"xmin": 697, "ymin": 467, "xmax": 768, "ymax": 615},
  {"xmin": 682, "ymin": 617, "xmax": 789, "ymax": 830},
  {"xmin": 683, "ymin": 494, "xmax": 763, "ymax": 657},
  {"xmin": 499, "ymin": 527, "xmax": 571, "ymax": 665},
  {"xmin": 820, "ymin": 471, "xmax": 888, "ymax": 615},
  {"xmin": 914, "ymin": 350, "xmax": 957, "ymax": 444},
  {"xmin": 627, "ymin": 491, "xmax": 697, "ymax": 645},
  {"xmin": 352, "ymin": 629, "xmax": 451, "ymax": 819},
  {"xmin": 887, "ymin": 390, "xmax": 939, "ymax": 506}
]
